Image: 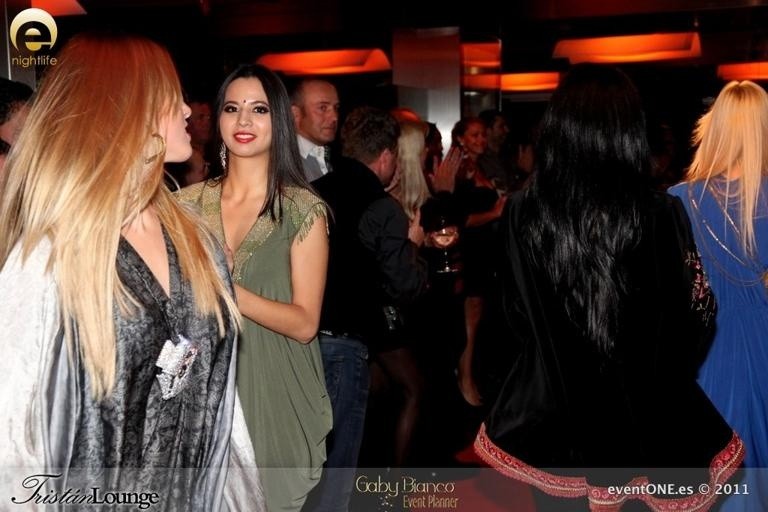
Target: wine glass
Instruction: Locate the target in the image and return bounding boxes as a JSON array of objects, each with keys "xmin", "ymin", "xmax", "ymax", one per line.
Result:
[{"xmin": 429, "ymin": 220, "xmax": 459, "ymax": 275}]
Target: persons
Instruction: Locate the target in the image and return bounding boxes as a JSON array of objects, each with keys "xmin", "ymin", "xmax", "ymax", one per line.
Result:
[{"xmin": 0, "ymin": 20, "xmax": 768, "ymax": 512}]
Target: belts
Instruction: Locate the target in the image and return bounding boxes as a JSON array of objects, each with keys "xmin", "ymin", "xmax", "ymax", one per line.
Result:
[{"xmin": 319, "ymin": 329, "xmax": 347, "ymax": 340}]
